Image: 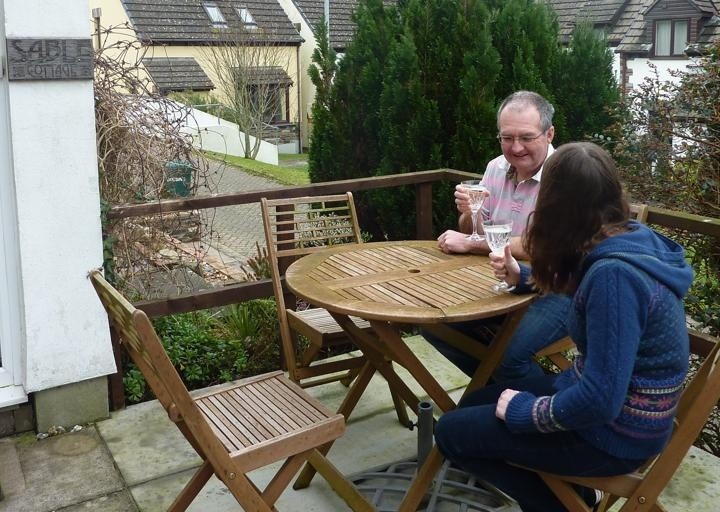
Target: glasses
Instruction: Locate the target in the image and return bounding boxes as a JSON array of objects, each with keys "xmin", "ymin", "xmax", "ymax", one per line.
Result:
[{"xmin": 496, "ymin": 129, "xmax": 545, "ymax": 144}]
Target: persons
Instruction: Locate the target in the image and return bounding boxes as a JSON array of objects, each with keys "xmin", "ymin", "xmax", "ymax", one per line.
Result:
[
  {"xmin": 423, "ymin": 89, "xmax": 572, "ymax": 388},
  {"xmin": 434, "ymin": 139, "xmax": 693, "ymax": 512}
]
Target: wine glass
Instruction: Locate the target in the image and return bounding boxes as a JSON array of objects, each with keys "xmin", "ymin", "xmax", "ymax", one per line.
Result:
[
  {"xmin": 462, "ymin": 180, "xmax": 488, "ymax": 243},
  {"xmin": 481, "ymin": 219, "xmax": 516, "ymax": 294}
]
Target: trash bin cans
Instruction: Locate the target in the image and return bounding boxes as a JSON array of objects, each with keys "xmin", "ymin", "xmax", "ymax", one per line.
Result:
[{"xmin": 164, "ymin": 162, "xmax": 191, "ymax": 197}]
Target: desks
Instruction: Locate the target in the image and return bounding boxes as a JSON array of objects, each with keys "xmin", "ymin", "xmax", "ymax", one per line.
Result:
[{"xmin": 285, "ymin": 236, "xmax": 540, "ymax": 512}]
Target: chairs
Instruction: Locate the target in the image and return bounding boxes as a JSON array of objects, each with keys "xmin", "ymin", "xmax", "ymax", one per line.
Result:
[
  {"xmin": 86, "ymin": 270, "xmax": 377, "ymax": 510},
  {"xmin": 259, "ymin": 191, "xmax": 409, "ymax": 429}
]
